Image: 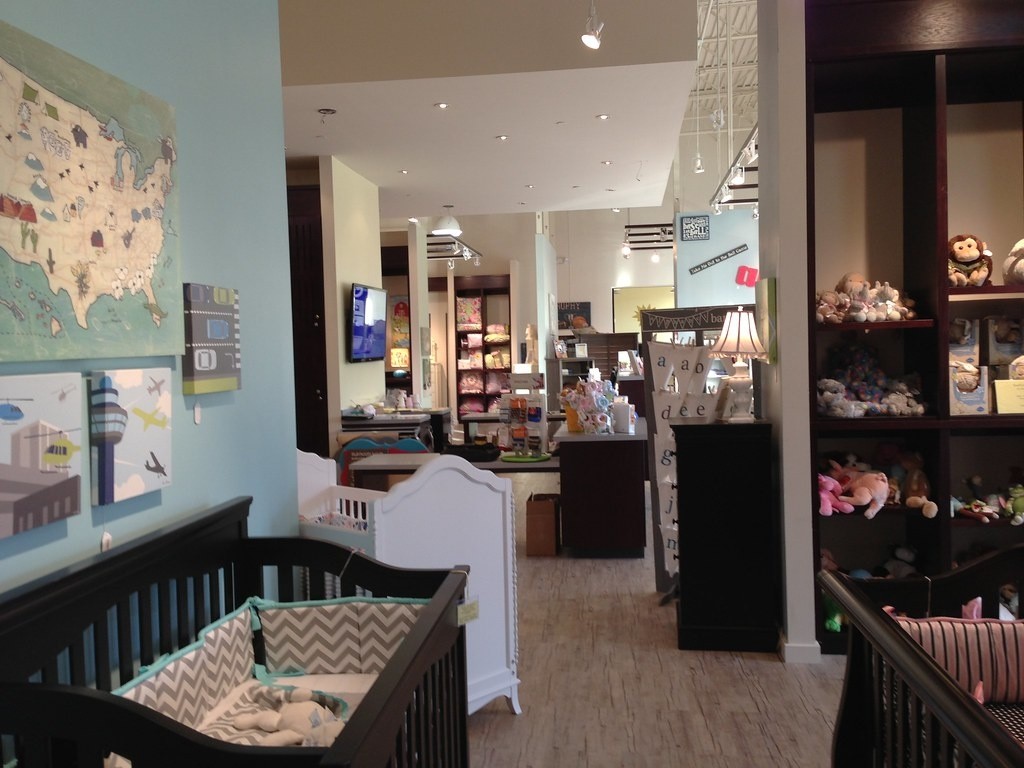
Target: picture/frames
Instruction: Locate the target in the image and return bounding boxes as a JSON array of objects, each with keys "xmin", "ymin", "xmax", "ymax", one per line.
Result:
[
  {"xmin": 551, "ymin": 340, "xmax": 567, "ymax": 358},
  {"xmin": 575, "ymin": 344, "xmax": 587, "ymax": 357}
]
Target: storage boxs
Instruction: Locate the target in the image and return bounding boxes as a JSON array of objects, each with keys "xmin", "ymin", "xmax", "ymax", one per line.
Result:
[
  {"xmin": 991, "ymin": 379, "xmax": 1024, "ymax": 414},
  {"xmin": 526, "ymin": 491, "xmax": 560, "ymax": 556},
  {"xmin": 981, "ymin": 314, "xmax": 1024, "ymax": 366},
  {"xmin": 613, "ymin": 404, "xmax": 635, "ymax": 435},
  {"xmin": 949, "ymin": 365, "xmax": 989, "ymax": 415},
  {"xmin": 949, "ymin": 317, "xmax": 980, "ymax": 366}
]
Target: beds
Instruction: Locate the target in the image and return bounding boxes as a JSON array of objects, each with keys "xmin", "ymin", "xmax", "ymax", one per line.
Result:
[
  {"xmin": 0, "ymin": 494, "xmax": 479, "ymax": 768},
  {"xmin": 818, "ymin": 543, "xmax": 1024, "ymax": 768},
  {"xmin": 296, "ymin": 447, "xmax": 522, "ymax": 715}
]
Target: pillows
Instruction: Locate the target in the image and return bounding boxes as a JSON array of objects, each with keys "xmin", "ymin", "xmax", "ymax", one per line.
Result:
[{"xmin": 883, "ymin": 603, "xmax": 1024, "ymax": 704}]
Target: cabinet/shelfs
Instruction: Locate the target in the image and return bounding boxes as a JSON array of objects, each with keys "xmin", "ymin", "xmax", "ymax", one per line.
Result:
[
  {"xmin": 578, "ymin": 332, "xmax": 640, "ymax": 381},
  {"xmin": 545, "ymin": 358, "xmax": 596, "ymax": 413},
  {"xmin": 554, "ymin": 416, "xmax": 649, "ymax": 559},
  {"xmin": 618, "ymin": 376, "xmax": 645, "ymax": 418},
  {"xmin": 391, "ymin": 408, "xmax": 451, "ymax": 452},
  {"xmin": 339, "ymin": 414, "xmax": 433, "ymax": 452},
  {"xmin": 665, "ymin": 415, "xmax": 781, "ymax": 653},
  {"xmin": 804, "ymin": 1, "xmax": 1024, "ymax": 654},
  {"xmin": 454, "ymin": 274, "xmax": 511, "ymax": 424}
]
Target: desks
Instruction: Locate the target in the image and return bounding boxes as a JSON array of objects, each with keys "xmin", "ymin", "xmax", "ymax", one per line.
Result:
[{"xmin": 350, "ymin": 454, "xmax": 559, "ymax": 529}]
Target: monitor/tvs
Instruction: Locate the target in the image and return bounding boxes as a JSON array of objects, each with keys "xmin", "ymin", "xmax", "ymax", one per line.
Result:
[{"xmin": 348, "ymin": 282, "xmax": 387, "ymax": 363}]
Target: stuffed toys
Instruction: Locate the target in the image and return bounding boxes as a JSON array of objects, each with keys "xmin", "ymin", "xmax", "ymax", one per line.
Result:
[
  {"xmin": 232, "ymin": 685, "xmax": 345, "ymax": 745},
  {"xmin": 812, "ymin": 233, "xmax": 1024, "ymax": 633}
]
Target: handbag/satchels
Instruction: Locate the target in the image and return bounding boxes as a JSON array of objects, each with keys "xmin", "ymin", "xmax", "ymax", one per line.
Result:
[{"xmin": 525, "ymin": 491, "xmax": 561, "ymax": 556}]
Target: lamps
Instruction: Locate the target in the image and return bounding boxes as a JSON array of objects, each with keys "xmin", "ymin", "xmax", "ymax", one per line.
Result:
[
  {"xmin": 391, "ymin": 348, "xmax": 409, "ymax": 378},
  {"xmin": 709, "ymin": 122, "xmax": 758, "ymax": 215},
  {"xmin": 432, "ymin": 216, "xmax": 462, "ymax": 237},
  {"xmin": 620, "ymin": 209, "xmax": 632, "ymax": 259},
  {"xmin": 580, "ymin": 0, "xmax": 605, "ymax": 50},
  {"xmin": 694, "ymin": 68, "xmax": 704, "ymax": 174},
  {"xmin": 447, "ymin": 241, "xmax": 480, "ymax": 270},
  {"xmin": 525, "ymin": 323, "xmax": 538, "ymax": 365},
  {"xmin": 707, "ymin": 306, "xmax": 768, "ymax": 423}
]
